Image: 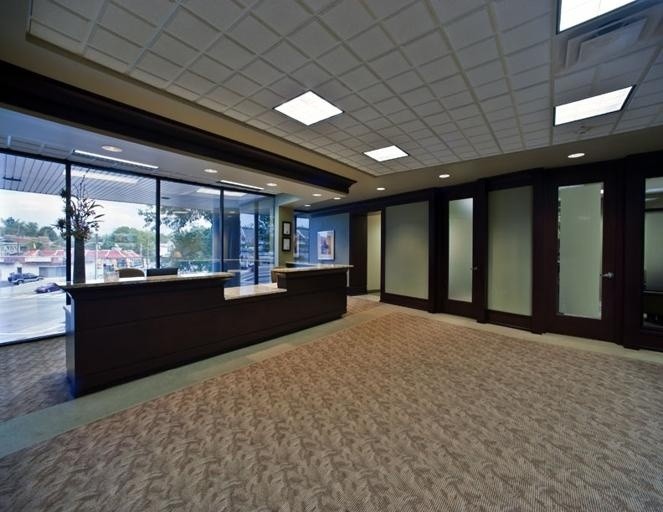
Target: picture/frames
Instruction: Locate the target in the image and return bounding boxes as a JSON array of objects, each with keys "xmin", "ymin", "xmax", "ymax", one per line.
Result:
[
  {"xmin": 282, "ymin": 222, "xmax": 291, "ymax": 251},
  {"xmin": 317, "ymin": 231, "xmax": 334, "ymax": 261}
]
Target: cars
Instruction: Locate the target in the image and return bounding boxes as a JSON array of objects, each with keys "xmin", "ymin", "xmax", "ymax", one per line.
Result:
[
  {"xmin": 36, "ymin": 282, "xmax": 61, "ymax": 293},
  {"xmin": 8, "ymin": 273, "xmax": 44, "ymax": 285}
]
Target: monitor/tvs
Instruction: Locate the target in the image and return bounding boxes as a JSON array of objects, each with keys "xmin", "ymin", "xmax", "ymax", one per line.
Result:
[{"xmin": 147, "ymin": 267, "xmax": 178, "ymax": 276}]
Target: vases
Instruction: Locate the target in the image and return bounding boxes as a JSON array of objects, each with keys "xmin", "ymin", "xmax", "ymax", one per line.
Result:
[{"xmin": 74, "ymin": 239, "xmax": 85, "ymax": 283}]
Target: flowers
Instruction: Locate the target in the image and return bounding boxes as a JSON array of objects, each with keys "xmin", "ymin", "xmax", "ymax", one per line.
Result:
[{"xmin": 49, "ymin": 173, "xmax": 107, "ymax": 239}]
